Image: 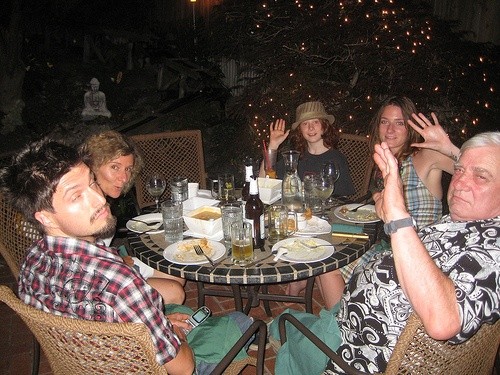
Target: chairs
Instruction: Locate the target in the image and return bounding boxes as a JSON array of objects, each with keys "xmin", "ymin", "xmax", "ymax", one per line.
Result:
[
  {"xmin": 0, "ymin": 285, "xmax": 266, "ymax": 375},
  {"xmin": 0, "ymin": 191, "xmax": 42, "ymax": 283},
  {"xmin": 334, "ymin": 134, "xmax": 375, "ymax": 205},
  {"xmin": 280, "ymin": 306, "xmax": 500, "ymax": 375},
  {"xmin": 129, "ymin": 130, "xmax": 206, "ymax": 208}
]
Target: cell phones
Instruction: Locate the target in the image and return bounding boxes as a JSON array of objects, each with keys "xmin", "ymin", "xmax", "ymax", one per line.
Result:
[{"xmin": 184, "ymin": 306, "xmax": 211, "ymax": 336}]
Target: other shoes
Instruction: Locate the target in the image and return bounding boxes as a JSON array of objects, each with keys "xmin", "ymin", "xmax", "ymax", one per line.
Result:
[{"xmin": 249, "ymin": 333, "xmax": 271, "ymax": 351}]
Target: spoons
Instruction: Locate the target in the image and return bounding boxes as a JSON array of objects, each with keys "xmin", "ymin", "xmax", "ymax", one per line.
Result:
[
  {"xmin": 127, "ymin": 217, "xmax": 159, "ymax": 227},
  {"xmin": 272, "ymin": 248, "xmax": 288, "ymax": 261}
]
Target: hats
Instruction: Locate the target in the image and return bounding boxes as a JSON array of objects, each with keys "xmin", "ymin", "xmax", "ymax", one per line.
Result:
[{"xmin": 292, "ymin": 100, "xmax": 336, "ymax": 132}]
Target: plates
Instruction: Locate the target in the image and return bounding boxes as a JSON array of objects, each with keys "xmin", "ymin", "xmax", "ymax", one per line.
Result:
[{"xmin": 126, "ymin": 190, "xmax": 381, "ymax": 265}]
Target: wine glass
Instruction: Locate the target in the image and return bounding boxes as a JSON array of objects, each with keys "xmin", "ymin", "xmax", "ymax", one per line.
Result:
[
  {"xmin": 145, "ymin": 173, "xmax": 166, "ymax": 215},
  {"xmin": 320, "ymin": 161, "xmax": 340, "ymax": 206},
  {"xmin": 310, "ymin": 173, "xmax": 334, "ymax": 220}
]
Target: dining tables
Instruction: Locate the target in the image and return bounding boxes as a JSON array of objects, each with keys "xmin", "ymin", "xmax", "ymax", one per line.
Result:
[{"xmin": 128, "ymin": 188, "xmax": 377, "ymax": 314}]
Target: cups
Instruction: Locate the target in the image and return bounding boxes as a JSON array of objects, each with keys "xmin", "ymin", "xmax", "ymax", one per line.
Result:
[
  {"xmin": 188, "ymin": 182, "xmax": 199, "ymax": 196},
  {"xmin": 211, "ymin": 173, "xmax": 235, "ymax": 201},
  {"xmin": 221, "ymin": 203, "xmax": 243, "ymax": 242},
  {"xmin": 160, "ymin": 200, "xmax": 183, "ymax": 244},
  {"xmin": 230, "ymin": 221, "xmax": 252, "ymax": 265},
  {"xmin": 303, "ymin": 176, "xmax": 322, "ymax": 213},
  {"xmin": 171, "ymin": 176, "xmax": 188, "ymax": 201},
  {"xmin": 265, "ymin": 203, "xmax": 297, "ymax": 244}
]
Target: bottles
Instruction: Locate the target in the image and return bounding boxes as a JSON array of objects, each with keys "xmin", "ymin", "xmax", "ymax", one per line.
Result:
[
  {"xmin": 281, "ymin": 150, "xmax": 304, "ymax": 211},
  {"xmin": 242, "ymin": 159, "xmax": 257, "ymax": 200},
  {"xmin": 245, "ymin": 175, "xmax": 269, "ymax": 247}
]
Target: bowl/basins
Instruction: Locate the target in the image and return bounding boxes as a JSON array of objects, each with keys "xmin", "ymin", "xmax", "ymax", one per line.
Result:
[
  {"xmin": 184, "ymin": 205, "xmax": 223, "ymax": 236},
  {"xmin": 257, "ymin": 177, "xmax": 282, "ymax": 201}
]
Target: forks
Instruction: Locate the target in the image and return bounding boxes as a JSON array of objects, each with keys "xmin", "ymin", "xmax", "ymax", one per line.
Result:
[
  {"xmin": 300, "ymin": 240, "xmax": 348, "ymax": 249},
  {"xmin": 193, "ymin": 245, "xmax": 214, "ymax": 268},
  {"xmin": 350, "ymin": 199, "xmax": 375, "ymax": 212}
]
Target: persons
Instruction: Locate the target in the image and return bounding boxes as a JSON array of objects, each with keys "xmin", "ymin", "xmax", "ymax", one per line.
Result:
[
  {"xmin": 82, "ymin": 78, "xmax": 111, "ymax": 118},
  {"xmin": 0, "ymin": 95, "xmax": 500, "ymax": 375}
]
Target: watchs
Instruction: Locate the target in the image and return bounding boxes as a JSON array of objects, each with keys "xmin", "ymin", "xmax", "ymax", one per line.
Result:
[{"xmin": 384, "ymin": 216, "xmax": 417, "ymax": 235}]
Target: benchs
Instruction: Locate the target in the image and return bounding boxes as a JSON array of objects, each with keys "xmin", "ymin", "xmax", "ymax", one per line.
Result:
[{"xmin": 27, "ymin": 89, "xmax": 238, "ymax": 137}]
